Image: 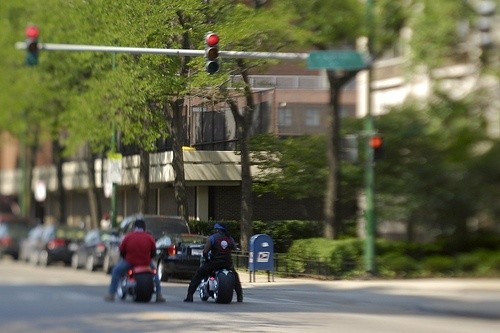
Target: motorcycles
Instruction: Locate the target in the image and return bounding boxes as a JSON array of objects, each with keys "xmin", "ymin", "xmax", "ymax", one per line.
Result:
[
  {"xmin": 199, "ymin": 253, "xmax": 235, "ymax": 303},
  {"xmin": 118, "ymin": 265, "xmax": 157, "ymax": 302}
]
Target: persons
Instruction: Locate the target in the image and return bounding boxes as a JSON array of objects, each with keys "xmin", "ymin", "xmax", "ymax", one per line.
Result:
[
  {"xmin": 103, "ymin": 219, "xmax": 168, "ymax": 303},
  {"xmin": 182, "ymin": 223, "xmax": 243, "ymax": 303}
]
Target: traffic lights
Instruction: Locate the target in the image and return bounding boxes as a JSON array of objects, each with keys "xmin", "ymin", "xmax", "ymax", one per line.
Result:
[
  {"xmin": 25, "ymin": 26, "xmax": 39, "ymax": 65},
  {"xmin": 205, "ymin": 29, "xmax": 220, "ymax": 74}
]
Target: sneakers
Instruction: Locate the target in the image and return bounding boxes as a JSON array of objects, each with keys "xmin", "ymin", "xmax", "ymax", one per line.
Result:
[
  {"xmin": 184, "ymin": 298, "xmax": 193, "ymax": 302},
  {"xmin": 237, "ymin": 297, "xmax": 243, "ymax": 302}
]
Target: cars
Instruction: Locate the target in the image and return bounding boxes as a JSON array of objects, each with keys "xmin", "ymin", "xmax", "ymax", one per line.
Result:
[
  {"xmin": 18, "ymin": 225, "xmax": 85, "ymax": 266},
  {"xmin": 151, "ymin": 233, "xmax": 209, "ymax": 281},
  {"xmin": 71, "ymin": 227, "xmax": 117, "ymax": 270}
]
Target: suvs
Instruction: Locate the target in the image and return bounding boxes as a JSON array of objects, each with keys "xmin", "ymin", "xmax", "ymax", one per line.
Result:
[{"xmin": 103, "ymin": 214, "xmax": 192, "ymax": 274}]
